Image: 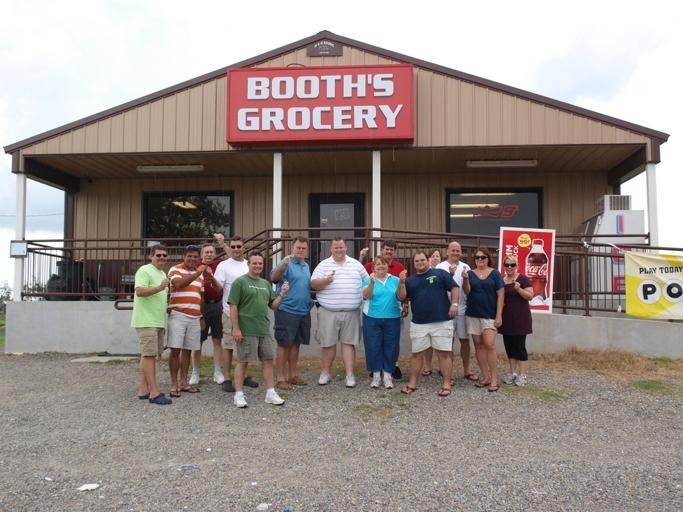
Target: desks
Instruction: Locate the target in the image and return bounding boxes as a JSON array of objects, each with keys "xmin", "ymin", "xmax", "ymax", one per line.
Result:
[{"xmin": 118, "ymin": 282, "xmax": 135, "ymax": 299}]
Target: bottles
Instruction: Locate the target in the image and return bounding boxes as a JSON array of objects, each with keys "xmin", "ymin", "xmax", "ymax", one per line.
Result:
[{"xmin": 524, "ymin": 238, "xmax": 550, "ymax": 311}]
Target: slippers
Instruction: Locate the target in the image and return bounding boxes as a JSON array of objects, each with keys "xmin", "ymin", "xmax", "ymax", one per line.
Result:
[
  {"xmin": 464, "ymin": 373, "xmax": 479, "ymax": 381},
  {"xmin": 488, "ymin": 384, "xmax": 500, "ymax": 392},
  {"xmin": 476, "ymin": 381, "xmax": 492, "ymax": 388},
  {"xmin": 401, "ymin": 385, "xmax": 418, "ymax": 395},
  {"xmin": 438, "ymin": 387, "xmax": 452, "ymax": 396},
  {"xmin": 450, "ymin": 379, "xmax": 455, "ymax": 386},
  {"xmin": 422, "ymin": 369, "xmax": 432, "ymax": 376}
]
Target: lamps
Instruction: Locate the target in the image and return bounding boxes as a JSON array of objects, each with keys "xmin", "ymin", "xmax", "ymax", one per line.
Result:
[
  {"xmin": 465, "ymin": 159, "xmax": 537, "ymax": 168},
  {"xmin": 171, "ymin": 199, "xmax": 199, "ymax": 210},
  {"xmin": 136, "ymin": 165, "xmax": 204, "ymax": 173}
]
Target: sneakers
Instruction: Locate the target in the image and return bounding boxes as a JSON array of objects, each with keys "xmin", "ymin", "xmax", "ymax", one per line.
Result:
[
  {"xmin": 370, "ymin": 377, "xmax": 383, "ymax": 388},
  {"xmin": 318, "ymin": 373, "xmax": 331, "ymax": 385},
  {"xmin": 290, "ymin": 376, "xmax": 307, "ymax": 386},
  {"xmin": 188, "ymin": 373, "xmax": 200, "ymax": 385},
  {"xmin": 514, "ymin": 374, "xmax": 528, "ymax": 387},
  {"xmin": 233, "ymin": 395, "xmax": 248, "ymax": 408},
  {"xmin": 214, "ymin": 373, "xmax": 225, "ymax": 385},
  {"xmin": 391, "ymin": 367, "xmax": 403, "ymax": 381},
  {"xmin": 503, "ymin": 373, "xmax": 518, "ymax": 385},
  {"xmin": 383, "ymin": 378, "xmax": 394, "ymax": 389},
  {"xmin": 345, "ymin": 375, "xmax": 356, "ymax": 387},
  {"xmin": 264, "ymin": 394, "xmax": 284, "ymax": 405},
  {"xmin": 275, "ymin": 381, "xmax": 292, "ymax": 391}
]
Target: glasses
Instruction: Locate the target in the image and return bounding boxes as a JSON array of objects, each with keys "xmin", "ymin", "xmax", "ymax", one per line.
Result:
[
  {"xmin": 153, "ymin": 254, "xmax": 168, "ymax": 258},
  {"xmin": 230, "ymin": 245, "xmax": 243, "ymax": 249},
  {"xmin": 474, "ymin": 256, "xmax": 489, "ymax": 259},
  {"xmin": 504, "ymin": 263, "xmax": 516, "ymax": 267}
]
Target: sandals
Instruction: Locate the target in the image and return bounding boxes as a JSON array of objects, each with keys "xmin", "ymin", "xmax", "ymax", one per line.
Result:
[
  {"xmin": 148, "ymin": 394, "xmax": 172, "ymax": 405},
  {"xmin": 243, "ymin": 377, "xmax": 258, "ymax": 388},
  {"xmin": 221, "ymin": 379, "xmax": 234, "ymax": 392},
  {"xmin": 169, "ymin": 390, "xmax": 181, "ymax": 397},
  {"xmin": 179, "ymin": 385, "xmax": 199, "ymax": 394},
  {"xmin": 139, "ymin": 393, "xmax": 165, "ymax": 400}
]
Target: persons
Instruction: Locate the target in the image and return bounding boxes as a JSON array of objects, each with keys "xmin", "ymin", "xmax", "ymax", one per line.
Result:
[
  {"xmin": 132, "ymin": 245, "xmax": 172, "ymax": 404},
  {"xmin": 497, "ymin": 255, "xmax": 534, "ymax": 387},
  {"xmin": 167, "ymin": 232, "xmax": 311, "ymax": 409},
  {"xmin": 359, "ymin": 241, "xmax": 506, "ymax": 397},
  {"xmin": 310, "ymin": 237, "xmax": 371, "ymax": 388}
]
